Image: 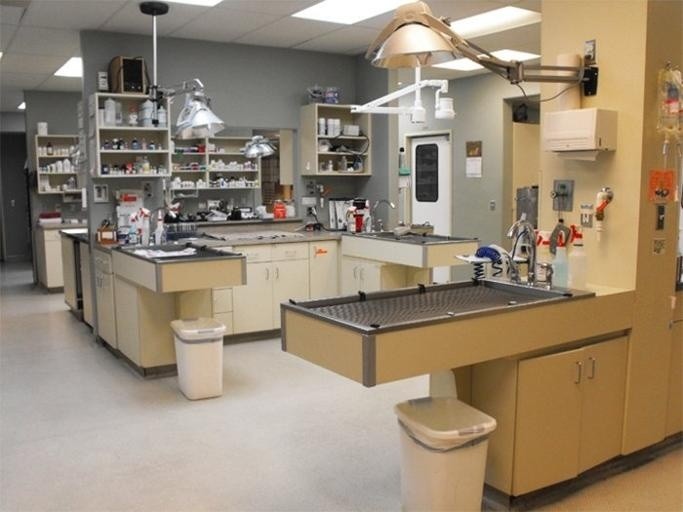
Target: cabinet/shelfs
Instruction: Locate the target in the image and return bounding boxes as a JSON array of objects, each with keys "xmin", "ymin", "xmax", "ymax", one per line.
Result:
[
  {"xmin": 234, "ymin": 242, "xmax": 310, "ymax": 336},
  {"xmin": 210, "ymin": 246, "xmax": 234, "ymax": 337},
  {"xmin": 166, "ymin": 134, "xmax": 262, "ymax": 190},
  {"xmin": 30, "ymin": 134, "xmax": 84, "ymax": 196},
  {"xmin": 87, "ymin": 92, "xmax": 172, "ymax": 180},
  {"xmin": 470, "ymin": 333, "xmax": 630, "ymax": 497},
  {"xmin": 339, "ymin": 254, "xmax": 407, "ymax": 298},
  {"xmin": 297, "ymin": 102, "xmax": 373, "ymax": 178},
  {"xmin": 308, "ymin": 240, "xmax": 338, "ymax": 301}
]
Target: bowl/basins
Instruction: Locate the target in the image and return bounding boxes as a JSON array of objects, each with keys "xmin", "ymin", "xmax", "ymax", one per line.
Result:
[{"xmin": 411, "ymin": 223, "xmax": 432, "ymax": 236}]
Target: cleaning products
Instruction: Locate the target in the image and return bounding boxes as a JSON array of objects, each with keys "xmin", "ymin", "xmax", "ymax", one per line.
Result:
[
  {"xmin": 345, "ymin": 207, "xmax": 356, "ymax": 235},
  {"xmin": 551, "ymin": 223, "xmax": 584, "ymax": 286},
  {"xmin": 127, "ymin": 207, "xmax": 165, "ymax": 247}
]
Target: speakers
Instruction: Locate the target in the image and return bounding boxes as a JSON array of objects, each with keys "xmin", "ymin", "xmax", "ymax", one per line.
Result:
[{"xmin": 111, "ymin": 56, "xmax": 146, "ymax": 95}]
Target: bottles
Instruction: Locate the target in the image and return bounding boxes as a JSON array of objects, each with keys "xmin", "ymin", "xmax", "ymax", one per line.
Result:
[
  {"xmin": 509, "ymin": 230, "xmax": 590, "ymax": 291},
  {"xmin": 37, "ymin": 97, "xmax": 375, "ymax": 246}
]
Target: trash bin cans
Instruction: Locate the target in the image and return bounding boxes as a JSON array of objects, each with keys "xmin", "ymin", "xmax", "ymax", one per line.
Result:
[
  {"xmin": 169, "ymin": 317, "xmax": 226, "ymax": 401},
  {"xmin": 394, "ymin": 394, "xmax": 497, "ymax": 512}
]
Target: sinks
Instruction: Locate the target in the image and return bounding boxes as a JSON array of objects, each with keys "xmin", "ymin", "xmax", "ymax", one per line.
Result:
[{"xmin": 356, "ymin": 231, "xmax": 394, "ymax": 238}]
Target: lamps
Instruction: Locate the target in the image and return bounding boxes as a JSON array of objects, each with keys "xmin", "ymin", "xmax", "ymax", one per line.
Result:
[
  {"xmin": 240, "ymin": 134, "xmax": 280, "ymax": 158},
  {"xmin": 348, "ymin": 0, "xmax": 598, "ymax": 96},
  {"xmin": 139, "ymin": 1, "xmax": 226, "ymax": 141}
]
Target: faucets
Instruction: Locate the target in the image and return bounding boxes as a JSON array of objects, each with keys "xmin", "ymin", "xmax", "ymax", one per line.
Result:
[
  {"xmin": 370, "ymin": 200, "xmax": 395, "ymax": 216},
  {"xmin": 507, "ymin": 220, "xmax": 536, "ymax": 284}
]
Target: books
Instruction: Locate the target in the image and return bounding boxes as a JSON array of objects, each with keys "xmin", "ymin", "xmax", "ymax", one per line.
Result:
[{"xmin": 132, "ymin": 246, "xmax": 198, "ymax": 258}]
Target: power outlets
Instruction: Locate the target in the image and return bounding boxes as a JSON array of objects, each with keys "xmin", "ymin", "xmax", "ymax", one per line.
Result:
[{"xmin": 551, "ymin": 179, "xmax": 575, "ymax": 211}]
